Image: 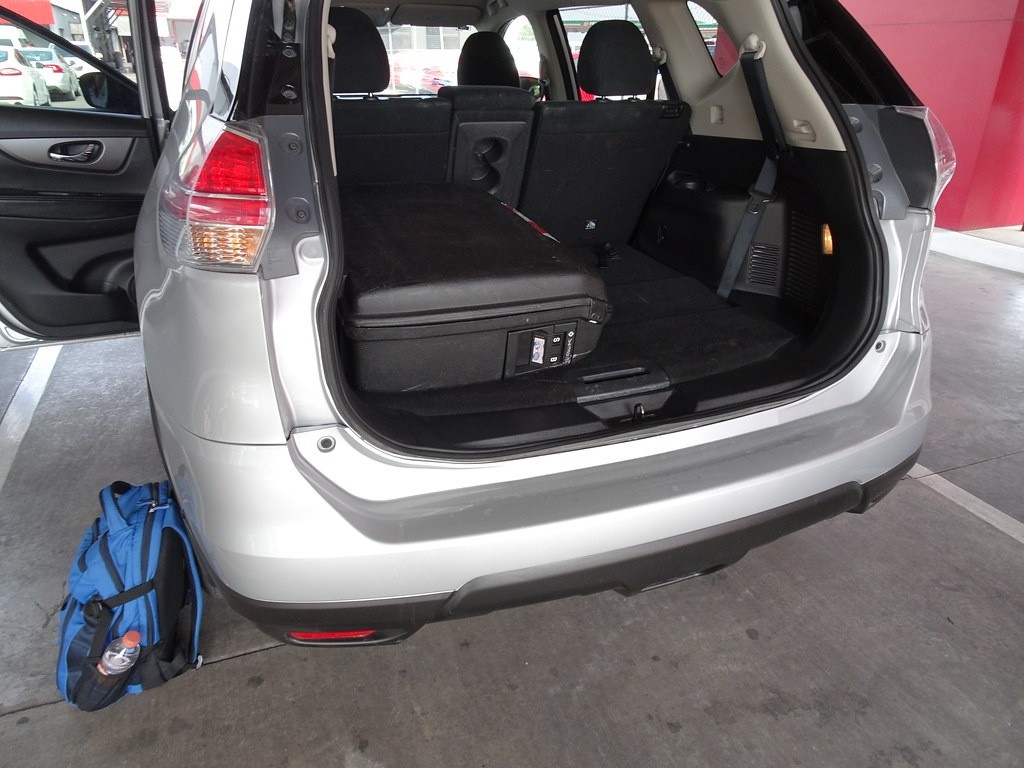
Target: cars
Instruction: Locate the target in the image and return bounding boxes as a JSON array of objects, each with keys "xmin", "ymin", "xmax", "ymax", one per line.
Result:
[
  {"xmin": 0, "ymin": 1, "xmax": 958, "ymax": 644},
  {"xmin": 0, "ymin": 25, "xmax": 104, "ymax": 108}
]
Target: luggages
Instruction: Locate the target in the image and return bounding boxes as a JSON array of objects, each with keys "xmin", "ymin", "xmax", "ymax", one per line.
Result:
[{"xmin": 337, "ymin": 190, "xmax": 615, "ymax": 395}]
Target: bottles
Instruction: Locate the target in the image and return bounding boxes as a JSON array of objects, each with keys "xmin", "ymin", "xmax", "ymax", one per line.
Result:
[{"xmin": 95, "ymin": 631, "xmax": 140, "ymax": 676}]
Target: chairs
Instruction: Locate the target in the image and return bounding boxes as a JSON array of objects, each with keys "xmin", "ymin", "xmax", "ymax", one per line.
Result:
[
  {"xmin": 325, "ymin": 6, "xmax": 452, "ymax": 191},
  {"xmin": 455, "ymin": 31, "xmax": 521, "ymax": 88},
  {"xmin": 519, "ymin": 21, "xmax": 692, "ymax": 251}
]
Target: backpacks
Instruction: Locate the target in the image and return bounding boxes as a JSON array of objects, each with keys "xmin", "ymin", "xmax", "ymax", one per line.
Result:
[{"xmin": 55, "ymin": 481, "xmax": 204, "ymax": 713}]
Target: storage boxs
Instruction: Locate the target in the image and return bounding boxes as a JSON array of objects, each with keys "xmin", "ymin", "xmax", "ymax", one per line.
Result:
[{"xmin": 334, "ymin": 181, "xmax": 612, "ymax": 392}]
